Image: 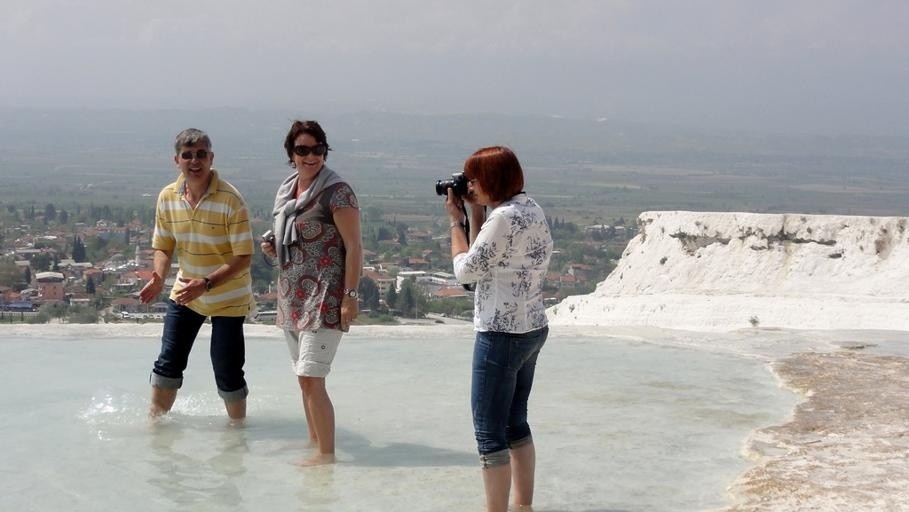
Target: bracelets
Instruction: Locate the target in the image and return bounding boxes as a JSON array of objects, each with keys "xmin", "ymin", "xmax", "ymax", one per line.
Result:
[{"xmin": 451, "ymin": 222, "xmax": 460, "ymax": 228}]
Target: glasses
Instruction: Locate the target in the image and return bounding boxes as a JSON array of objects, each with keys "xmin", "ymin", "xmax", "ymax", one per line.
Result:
[
  {"xmin": 182, "ymin": 150, "xmax": 207, "ymax": 159},
  {"xmin": 293, "ymin": 144, "xmax": 327, "ymax": 156},
  {"xmin": 467, "ymin": 179, "xmax": 479, "ymax": 187}
]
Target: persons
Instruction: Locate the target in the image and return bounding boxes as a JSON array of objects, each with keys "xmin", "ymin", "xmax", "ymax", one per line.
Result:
[
  {"xmin": 261, "ymin": 121, "xmax": 363, "ymax": 466},
  {"xmin": 445, "ymin": 146, "xmax": 553, "ymax": 512},
  {"xmin": 139, "ymin": 128, "xmax": 256, "ymax": 419}
]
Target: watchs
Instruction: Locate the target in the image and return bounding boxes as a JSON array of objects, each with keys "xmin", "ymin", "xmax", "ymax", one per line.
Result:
[
  {"xmin": 203, "ymin": 278, "xmax": 213, "ymax": 289},
  {"xmin": 343, "ymin": 288, "xmax": 358, "ymax": 298}
]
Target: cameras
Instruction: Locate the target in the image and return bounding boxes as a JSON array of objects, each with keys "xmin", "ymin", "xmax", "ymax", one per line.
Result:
[
  {"xmin": 263, "ymin": 230, "xmax": 275, "ymax": 247},
  {"xmin": 436, "ymin": 172, "xmax": 473, "ymax": 196}
]
